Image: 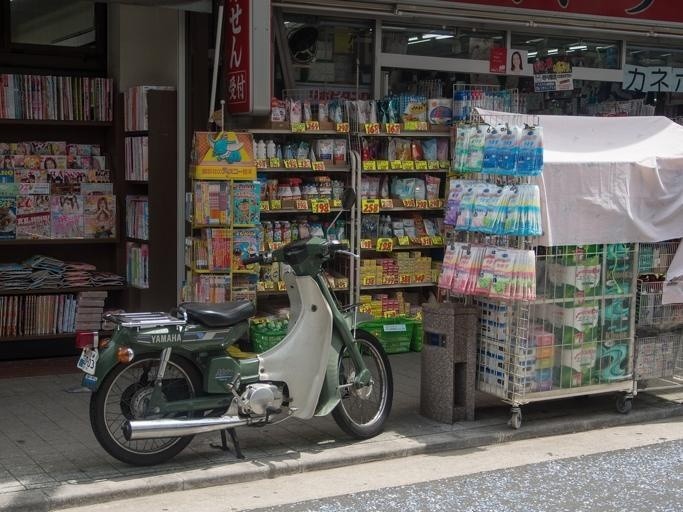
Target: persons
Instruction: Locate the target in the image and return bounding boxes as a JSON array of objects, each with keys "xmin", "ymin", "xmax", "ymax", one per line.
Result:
[{"xmin": 510, "ymin": 51, "xmax": 525, "ymax": 75}]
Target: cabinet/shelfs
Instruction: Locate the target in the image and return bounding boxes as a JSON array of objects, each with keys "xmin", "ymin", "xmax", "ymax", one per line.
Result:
[
  {"xmin": 439, "ymin": 232, "xmax": 641, "ymax": 431},
  {"xmin": 233, "ymin": 105, "xmax": 355, "ymax": 346},
  {"xmin": 120, "ymin": 87, "xmax": 171, "ymax": 322},
  {"xmin": 355, "ymin": 97, "xmax": 455, "ymax": 334},
  {"xmin": 2, "ymin": 77, "xmax": 117, "ymax": 352}
]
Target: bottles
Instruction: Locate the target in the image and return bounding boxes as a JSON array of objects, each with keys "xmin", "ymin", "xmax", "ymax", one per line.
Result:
[{"xmin": 255, "ymin": 140, "xmax": 277, "ymax": 160}]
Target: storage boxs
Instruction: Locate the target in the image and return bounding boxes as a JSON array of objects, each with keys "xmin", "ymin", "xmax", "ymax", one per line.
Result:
[{"xmin": 534, "ymin": 260, "xmax": 602, "ymax": 386}]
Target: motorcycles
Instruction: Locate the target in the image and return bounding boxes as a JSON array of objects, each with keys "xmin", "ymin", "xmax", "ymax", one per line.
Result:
[{"xmin": 60, "ymin": 187, "xmax": 394, "ymax": 466}]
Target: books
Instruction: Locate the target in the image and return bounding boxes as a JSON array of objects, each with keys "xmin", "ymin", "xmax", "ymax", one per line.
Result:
[{"xmin": 1, "ymin": 72, "xmax": 177, "ymax": 338}]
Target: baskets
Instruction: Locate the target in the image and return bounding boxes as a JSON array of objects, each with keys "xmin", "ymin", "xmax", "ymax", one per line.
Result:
[
  {"xmin": 250, "ymin": 323, "xmax": 290, "ymax": 352},
  {"xmin": 357, "ymin": 318, "xmax": 422, "ymax": 355}
]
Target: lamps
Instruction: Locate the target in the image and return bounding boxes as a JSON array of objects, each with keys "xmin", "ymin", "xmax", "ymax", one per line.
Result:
[
  {"xmin": 408, "ymin": 33, "xmax": 455, "ymax": 45},
  {"xmin": 525, "ymin": 37, "xmax": 615, "ymax": 59}
]
